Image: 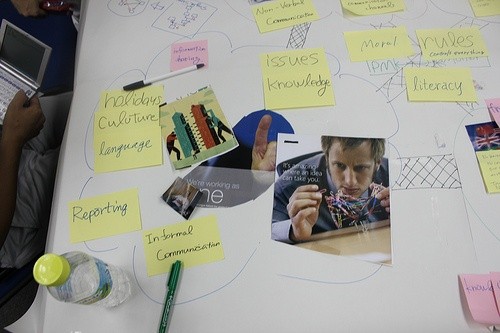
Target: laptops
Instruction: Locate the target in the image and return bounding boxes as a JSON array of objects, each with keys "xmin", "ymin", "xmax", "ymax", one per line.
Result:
[{"xmin": 0, "ymin": 19, "xmax": 52, "ymax": 126}]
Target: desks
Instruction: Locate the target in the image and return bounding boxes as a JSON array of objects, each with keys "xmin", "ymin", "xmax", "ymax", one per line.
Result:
[
  {"xmin": 290, "ymin": 218, "xmax": 392, "ymax": 264},
  {"xmin": 3, "ymin": 0, "xmax": 499, "ymax": 333}
]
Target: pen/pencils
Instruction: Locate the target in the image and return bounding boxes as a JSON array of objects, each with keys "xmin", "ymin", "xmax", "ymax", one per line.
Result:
[
  {"xmin": 159, "ymin": 260, "xmax": 181, "ymax": 333},
  {"xmin": 123, "ymin": 63, "xmax": 204, "ymax": 91}
]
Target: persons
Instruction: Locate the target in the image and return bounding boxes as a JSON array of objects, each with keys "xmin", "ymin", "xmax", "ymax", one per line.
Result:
[
  {"xmin": 0, "ymin": 88, "xmax": 44, "ymax": 272},
  {"xmin": 170, "ymin": 191, "xmax": 191, "ymax": 211},
  {"xmin": 190, "ymin": 114, "xmax": 277, "ymax": 206},
  {"xmin": 272, "ymin": 136, "xmax": 390, "ymax": 244}
]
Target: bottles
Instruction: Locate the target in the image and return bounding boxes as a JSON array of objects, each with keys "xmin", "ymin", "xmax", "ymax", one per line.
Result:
[{"xmin": 33, "ymin": 250, "xmax": 131, "ymax": 308}]
